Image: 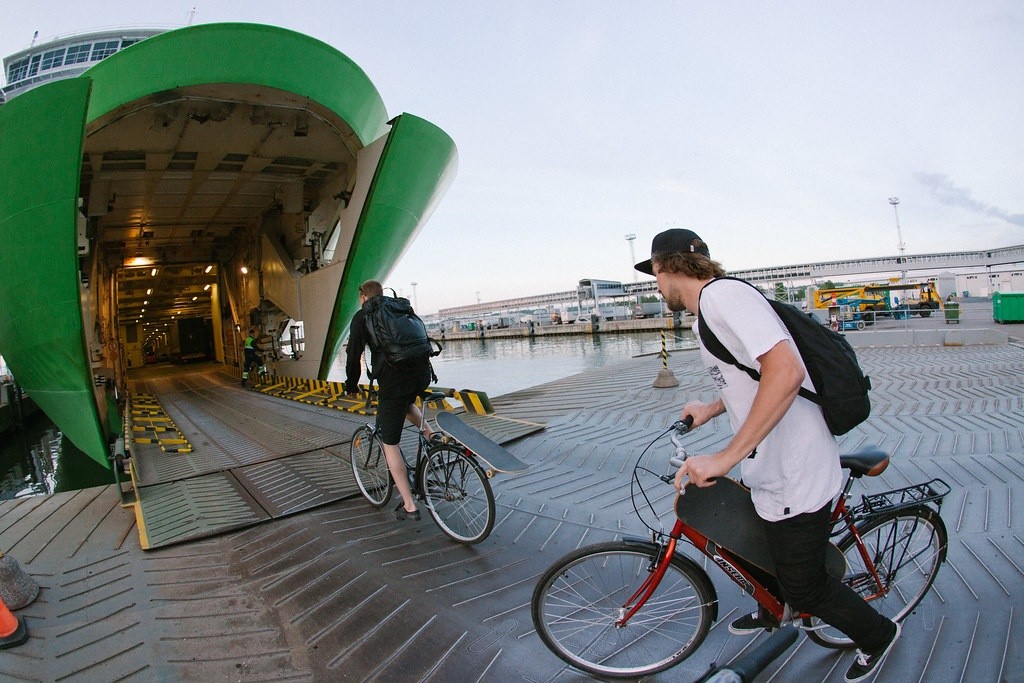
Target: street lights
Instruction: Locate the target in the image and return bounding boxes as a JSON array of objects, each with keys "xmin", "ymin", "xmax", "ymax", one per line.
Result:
[
  {"xmin": 624, "ymin": 232, "xmax": 640, "ymax": 302},
  {"xmin": 410, "ymin": 282, "xmax": 418, "ymax": 314},
  {"xmin": 889, "ymin": 193, "xmax": 908, "ymax": 301}
]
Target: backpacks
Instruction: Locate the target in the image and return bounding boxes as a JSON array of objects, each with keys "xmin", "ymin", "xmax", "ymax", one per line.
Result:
[
  {"xmin": 698, "ymin": 276, "xmax": 872, "ymax": 436},
  {"xmin": 362, "ymin": 287, "xmax": 432, "ymax": 366}
]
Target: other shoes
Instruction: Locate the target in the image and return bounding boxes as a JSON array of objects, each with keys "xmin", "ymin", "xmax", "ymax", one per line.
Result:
[{"xmin": 241, "ymin": 382, "xmax": 246, "ymax": 387}]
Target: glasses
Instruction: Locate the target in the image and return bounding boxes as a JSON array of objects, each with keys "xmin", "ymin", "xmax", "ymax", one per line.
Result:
[{"xmin": 359, "ymin": 286, "xmax": 366, "ymax": 298}]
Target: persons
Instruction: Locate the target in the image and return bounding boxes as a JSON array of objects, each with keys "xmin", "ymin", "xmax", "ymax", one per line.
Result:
[
  {"xmin": 343, "ymin": 280, "xmax": 442, "ymax": 522},
  {"xmin": 241, "ymin": 330, "xmax": 268, "ymax": 386},
  {"xmin": 634, "ymin": 229, "xmax": 901, "ymax": 683}
]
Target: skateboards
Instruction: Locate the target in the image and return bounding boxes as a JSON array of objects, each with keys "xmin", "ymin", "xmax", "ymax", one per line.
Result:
[
  {"xmin": 674, "ymin": 474, "xmax": 847, "ymax": 582},
  {"xmin": 435, "ymin": 411, "xmax": 530, "ymax": 479}
]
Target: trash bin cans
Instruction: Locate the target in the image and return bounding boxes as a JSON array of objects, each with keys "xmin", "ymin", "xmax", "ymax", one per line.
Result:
[
  {"xmin": 963, "ymin": 291, "xmax": 968, "ymax": 298},
  {"xmin": 943, "ymin": 302, "xmax": 959, "ymax": 324},
  {"xmin": 468, "ymin": 323, "xmax": 475, "ymax": 331},
  {"xmin": 992, "ymin": 290, "xmax": 1024, "ymax": 325}
]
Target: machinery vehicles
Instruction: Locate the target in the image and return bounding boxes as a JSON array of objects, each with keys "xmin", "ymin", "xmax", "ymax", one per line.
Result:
[
  {"xmin": 891, "ymin": 282, "xmax": 944, "ymax": 320},
  {"xmin": 800, "ymin": 283, "xmax": 892, "ymax": 333}
]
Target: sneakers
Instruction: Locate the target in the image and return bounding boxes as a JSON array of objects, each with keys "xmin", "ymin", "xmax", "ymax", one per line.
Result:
[
  {"xmin": 844, "ymin": 622, "xmax": 901, "ymax": 683},
  {"xmin": 391, "ymin": 504, "xmax": 421, "ymax": 521},
  {"xmin": 728, "ymin": 610, "xmax": 764, "ymax": 634}
]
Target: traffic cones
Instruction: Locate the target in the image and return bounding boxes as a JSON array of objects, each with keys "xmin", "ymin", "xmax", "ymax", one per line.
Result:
[{"xmin": 0, "ymin": 594, "xmax": 31, "ymax": 649}]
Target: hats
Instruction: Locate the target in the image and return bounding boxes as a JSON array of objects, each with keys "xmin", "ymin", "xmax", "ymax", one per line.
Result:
[{"xmin": 634, "ymin": 228, "xmax": 710, "ymax": 276}]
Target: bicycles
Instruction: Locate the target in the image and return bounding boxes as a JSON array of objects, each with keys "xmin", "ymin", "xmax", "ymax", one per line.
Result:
[
  {"xmin": 344, "ymin": 388, "xmax": 496, "ymax": 545},
  {"xmin": 530, "ymin": 414, "xmax": 954, "ymax": 677}
]
